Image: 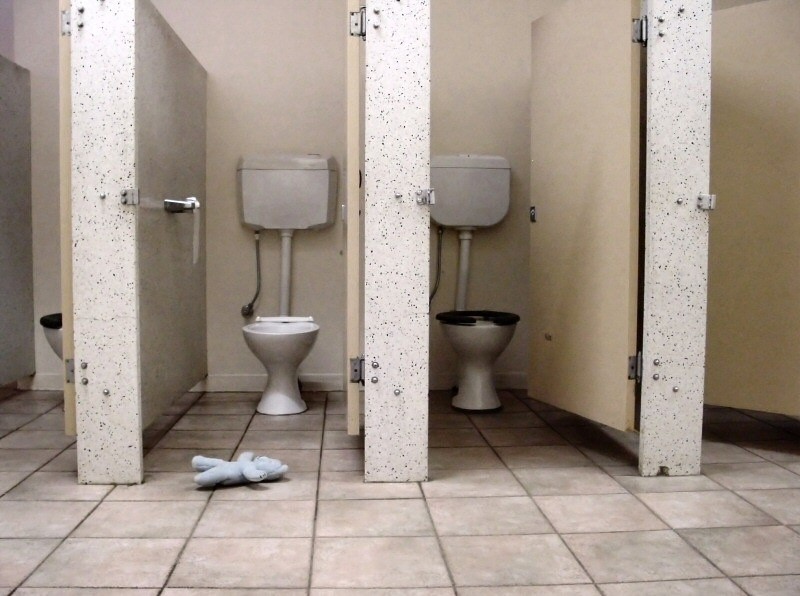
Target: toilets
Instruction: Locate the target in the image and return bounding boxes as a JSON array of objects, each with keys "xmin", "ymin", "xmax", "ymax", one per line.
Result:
[
  {"xmin": 39, "ymin": 313, "xmax": 64, "ymax": 359},
  {"xmin": 430, "ymin": 154, "xmax": 522, "ymax": 417},
  {"xmin": 235, "ymin": 151, "xmax": 338, "ymax": 417}
]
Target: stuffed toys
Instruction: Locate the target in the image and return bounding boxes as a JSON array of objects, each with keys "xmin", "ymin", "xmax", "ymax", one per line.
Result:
[{"xmin": 192, "ymin": 450, "xmax": 289, "ymax": 489}]
